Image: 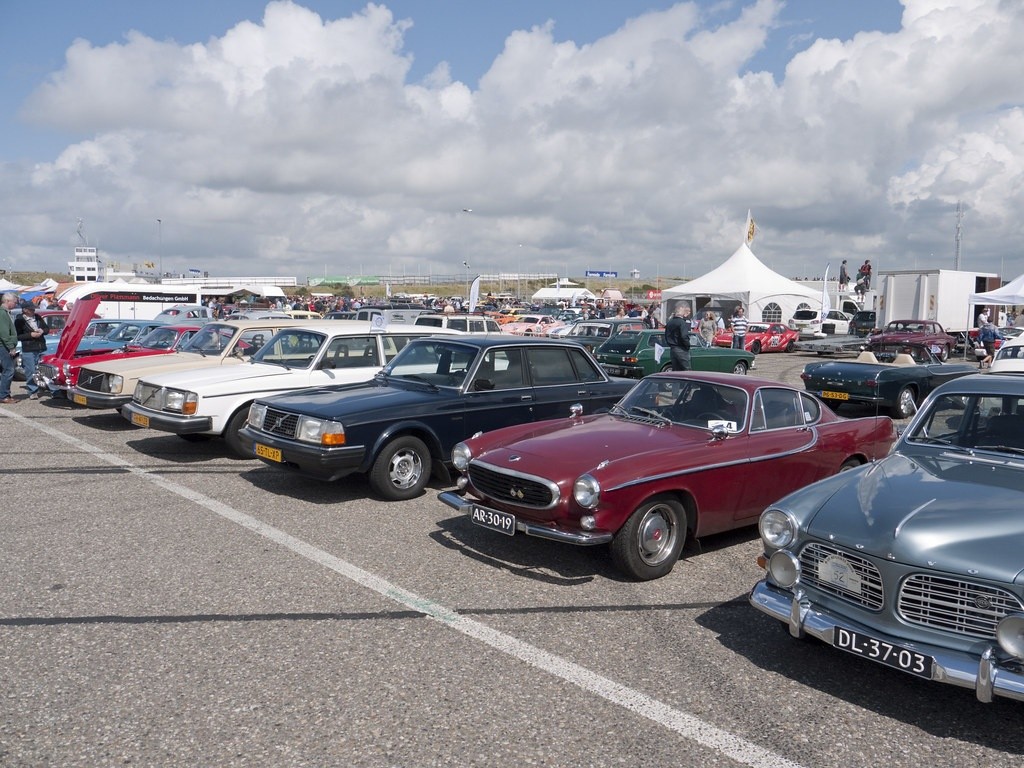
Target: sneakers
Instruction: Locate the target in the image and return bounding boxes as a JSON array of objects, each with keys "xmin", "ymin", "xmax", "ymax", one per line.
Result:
[{"xmin": 29, "ymin": 392, "xmax": 39, "ymax": 400}]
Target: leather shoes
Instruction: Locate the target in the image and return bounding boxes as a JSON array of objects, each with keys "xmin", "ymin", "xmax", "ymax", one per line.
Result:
[{"xmin": 0, "ymin": 396, "xmax": 21, "ymax": 404}]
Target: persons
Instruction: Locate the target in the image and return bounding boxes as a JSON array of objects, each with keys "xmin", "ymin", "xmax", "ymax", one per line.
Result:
[
  {"xmin": 838, "ymin": 259, "xmax": 872, "ymax": 302},
  {"xmin": 0, "ymin": 292, "xmax": 20, "ymax": 404},
  {"xmin": 665, "ymin": 301, "xmax": 694, "ymax": 400},
  {"xmin": 20, "ymin": 295, "xmax": 77, "ymax": 331},
  {"xmin": 201, "ymin": 290, "xmax": 644, "ymax": 327},
  {"xmin": 977, "ymin": 305, "xmax": 1024, "ymax": 369},
  {"xmin": 14, "ymin": 301, "xmax": 49, "ymax": 400},
  {"xmin": 642, "ymin": 301, "xmax": 750, "ymax": 349}
]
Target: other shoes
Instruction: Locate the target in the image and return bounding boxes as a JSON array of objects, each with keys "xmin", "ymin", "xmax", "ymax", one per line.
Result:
[
  {"xmin": 987, "ymin": 366, "xmax": 991, "ymax": 369},
  {"xmin": 980, "ymin": 360, "xmax": 984, "ymax": 369}
]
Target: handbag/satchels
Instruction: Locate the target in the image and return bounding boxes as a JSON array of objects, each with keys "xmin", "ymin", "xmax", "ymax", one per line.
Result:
[
  {"xmin": 856, "ymin": 272, "xmax": 863, "ymax": 280},
  {"xmin": 856, "ymin": 276, "xmax": 866, "ymax": 285}
]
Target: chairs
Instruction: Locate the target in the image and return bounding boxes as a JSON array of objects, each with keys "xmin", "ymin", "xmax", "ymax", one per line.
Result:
[
  {"xmin": 362, "ymin": 345, "xmax": 377, "ymax": 366},
  {"xmin": 977, "ymin": 416, "xmax": 1023, "ymax": 450},
  {"xmin": 505, "ymin": 360, "xmax": 533, "ymax": 385},
  {"xmin": 688, "ymin": 390, "xmax": 715, "ymax": 416},
  {"xmin": 333, "ymin": 345, "xmax": 348, "ymax": 366},
  {"xmin": 857, "ymin": 351, "xmax": 878, "ymax": 365},
  {"xmin": 893, "ymin": 354, "xmax": 915, "ymax": 366},
  {"xmin": 466, "ymin": 354, "xmax": 489, "ymax": 383}
]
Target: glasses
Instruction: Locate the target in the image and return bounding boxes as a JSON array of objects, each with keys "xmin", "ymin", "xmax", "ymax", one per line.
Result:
[{"xmin": 705, "ymin": 313, "xmax": 709, "ymax": 316}]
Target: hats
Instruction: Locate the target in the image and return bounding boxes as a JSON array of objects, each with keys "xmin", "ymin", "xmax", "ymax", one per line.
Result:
[{"xmin": 21, "ymin": 301, "xmax": 37, "ymax": 310}]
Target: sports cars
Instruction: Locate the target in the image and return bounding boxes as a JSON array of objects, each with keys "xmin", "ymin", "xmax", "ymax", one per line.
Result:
[{"xmin": 800, "ymin": 340, "xmax": 981, "ymax": 419}]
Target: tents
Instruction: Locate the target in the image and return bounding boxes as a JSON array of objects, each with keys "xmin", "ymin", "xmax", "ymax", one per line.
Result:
[
  {"xmin": 660, "ymin": 242, "xmax": 829, "ymax": 339},
  {"xmin": 967, "ymin": 274, "xmax": 1024, "ymax": 322},
  {"xmin": 0, "ymin": 278, "xmax": 60, "ymax": 303}
]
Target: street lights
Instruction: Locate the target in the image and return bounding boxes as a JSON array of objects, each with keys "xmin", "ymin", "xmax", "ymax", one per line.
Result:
[
  {"xmin": 463, "ymin": 261, "xmax": 471, "ymax": 312},
  {"xmin": 157, "ymin": 219, "xmax": 162, "ymax": 284}
]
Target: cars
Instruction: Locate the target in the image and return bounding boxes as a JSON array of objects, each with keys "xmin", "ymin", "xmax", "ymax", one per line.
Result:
[
  {"xmin": 72, "ymin": 312, "xmax": 415, "ymax": 415},
  {"xmin": 436, "ymin": 371, "xmax": 898, "ymax": 582},
  {"xmin": 238, "ymin": 334, "xmax": 661, "ymax": 501},
  {"xmin": 858, "ymin": 319, "xmax": 957, "ymax": 363},
  {"xmin": 120, "ymin": 316, "xmax": 510, "ymax": 461},
  {"xmin": 16, "ymin": 287, "xmax": 877, "ymax": 400},
  {"xmin": 969, "ymin": 325, "xmax": 1024, "ymax": 375},
  {"xmin": 747, "ymin": 374, "xmax": 1024, "ymax": 703}
]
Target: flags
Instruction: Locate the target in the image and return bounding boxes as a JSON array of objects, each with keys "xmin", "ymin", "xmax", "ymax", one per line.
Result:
[{"xmin": 744, "ymin": 212, "xmax": 760, "ymax": 247}]
